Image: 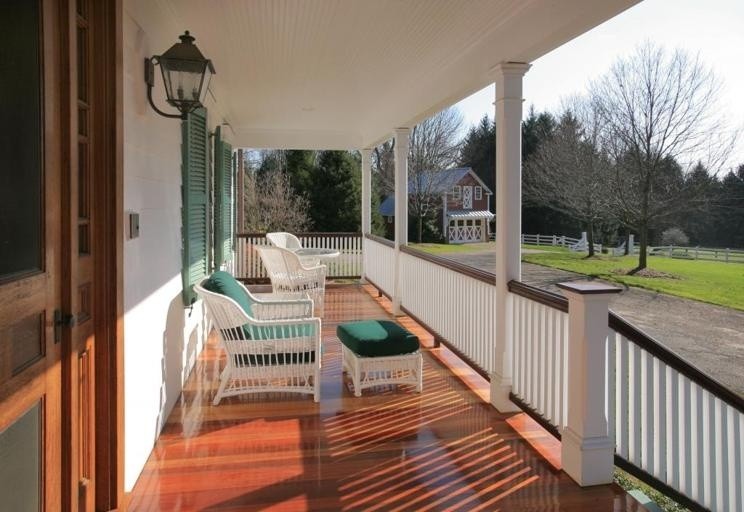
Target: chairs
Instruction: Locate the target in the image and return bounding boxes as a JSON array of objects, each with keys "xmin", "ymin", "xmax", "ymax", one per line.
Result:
[
  {"xmin": 193, "ymin": 270, "xmax": 322, "ymax": 406},
  {"xmin": 251, "ymin": 246, "xmax": 327, "ymax": 319},
  {"xmin": 265, "ymin": 232, "xmax": 302, "ymax": 253}
]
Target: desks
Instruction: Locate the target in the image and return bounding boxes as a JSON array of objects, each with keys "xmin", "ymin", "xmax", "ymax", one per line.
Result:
[{"xmin": 293, "ymin": 248, "xmax": 339, "ymax": 268}]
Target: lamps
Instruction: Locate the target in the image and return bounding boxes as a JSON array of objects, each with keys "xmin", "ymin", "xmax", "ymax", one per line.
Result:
[{"xmin": 144, "ymin": 30, "xmax": 215, "ymax": 120}]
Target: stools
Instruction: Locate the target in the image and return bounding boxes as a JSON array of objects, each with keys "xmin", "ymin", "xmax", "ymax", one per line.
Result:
[{"xmin": 336, "ymin": 319, "xmax": 423, "ymax": 397}]
